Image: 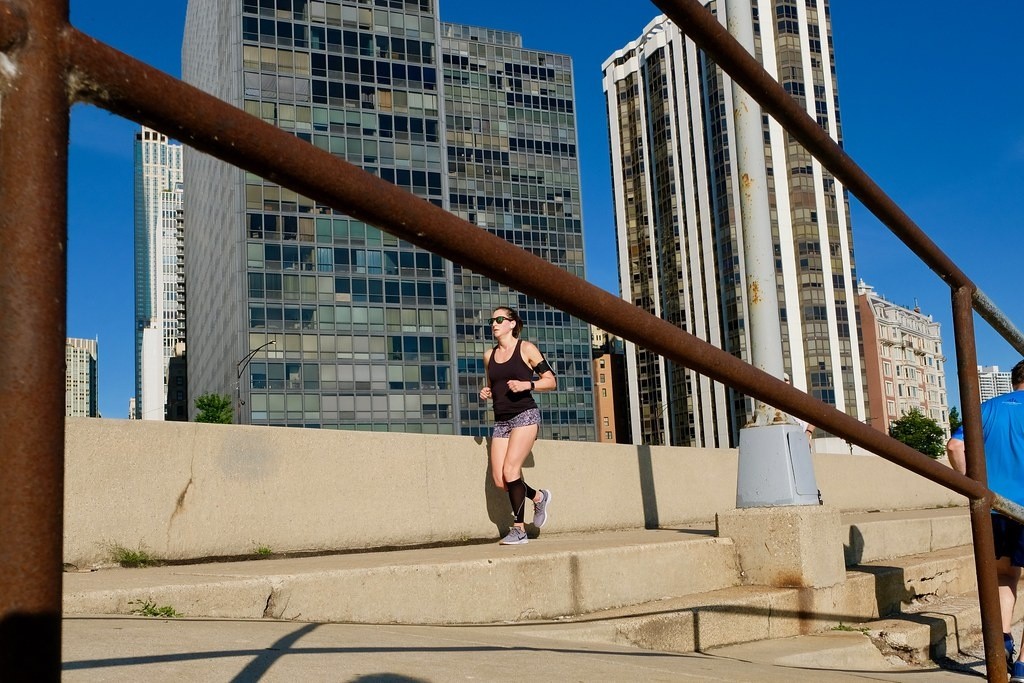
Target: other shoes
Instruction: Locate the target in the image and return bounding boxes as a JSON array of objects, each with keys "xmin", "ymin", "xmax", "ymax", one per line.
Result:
[
  {"xmin": 1010, "ymin": 662, "xmax": 1024, "ymax": 683},
  {"xmin": 1003, "ymin": 632, "xmax": 1017, "ymax": 677}
]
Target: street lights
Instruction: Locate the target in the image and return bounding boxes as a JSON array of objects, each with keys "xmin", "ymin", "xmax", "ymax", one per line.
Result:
[{"xmin": 236, "ymin": 339, "xmax": 277, "ymax": 423}]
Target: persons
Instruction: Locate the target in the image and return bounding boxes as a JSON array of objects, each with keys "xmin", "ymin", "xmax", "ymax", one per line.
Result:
[
  {"xmin": 947, "ymin": 361, "xmax": 1024, "ymax": 683},
  {"xmin": 784, "ymin": 374, "xmax": 816, "ymax": 451},
  {"xmin": 480, "ymin": 307, "xmax": 558, "ymax": 545}
]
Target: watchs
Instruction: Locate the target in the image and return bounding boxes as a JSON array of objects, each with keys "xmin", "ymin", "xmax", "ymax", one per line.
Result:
[{"xmin": 530, "ymin": 381, "xmax": 535, "ymax": 391}]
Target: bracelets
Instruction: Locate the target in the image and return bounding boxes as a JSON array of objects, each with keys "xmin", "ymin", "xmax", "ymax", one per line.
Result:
[{"xmin": 806, "ymin": 429, "xmax": 813, "ymax": 434}]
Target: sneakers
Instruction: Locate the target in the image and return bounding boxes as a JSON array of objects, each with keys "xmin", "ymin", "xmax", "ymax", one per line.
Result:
[
  {"xmin": 499, "ymin": 526, "xmax": 529, "ymax": 544},
  {"xmin": 533, "ymin": 489, "xmax": 552, "ymax": 528}
]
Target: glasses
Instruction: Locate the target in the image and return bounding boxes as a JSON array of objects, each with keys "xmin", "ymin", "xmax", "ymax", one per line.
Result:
[{"xmin": 487, "ymin": 315, "xmax": 516, "ymax": 326}]
